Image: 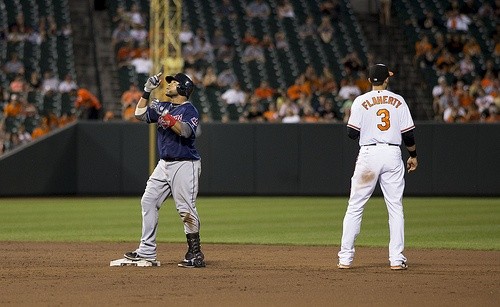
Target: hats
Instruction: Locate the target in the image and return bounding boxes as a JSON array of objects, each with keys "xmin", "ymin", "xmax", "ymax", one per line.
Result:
[{"xmin": 369, "ymin": 64, "xmax": 393, "ymax": 82}]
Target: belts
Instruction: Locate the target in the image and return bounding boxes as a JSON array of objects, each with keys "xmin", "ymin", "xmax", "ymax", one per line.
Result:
[{"xmin": 364, "ymin": 143, "xmax": 399, "ymax": 146}]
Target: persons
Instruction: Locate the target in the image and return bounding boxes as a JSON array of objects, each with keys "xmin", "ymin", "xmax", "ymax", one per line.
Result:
[
  {"xmin": 413, "ymin": 8, "xmax": 500, "ymax": 125},
  {"xmin": 125, "ymin": 71, "xmax": 205, "ymax": 269},
  {"xmin": 337, "ymin": 62, "xmax": 418, "ymax": 271},
  {"xmin": 0, "ymin": 10, "xmax": 115, "ymax": 155},
  {"xmin": 113, "ymin": 3, "xmax": 373, "ymax": 125}
]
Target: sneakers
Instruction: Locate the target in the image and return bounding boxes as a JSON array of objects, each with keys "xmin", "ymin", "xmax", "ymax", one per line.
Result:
[
  {"xmin": 178, "ymin": 252, "xmax": 204, "ymax": 268},
  {"xmin": 124, "ymin": 251, "xmax": 157, "ymax": 260}
]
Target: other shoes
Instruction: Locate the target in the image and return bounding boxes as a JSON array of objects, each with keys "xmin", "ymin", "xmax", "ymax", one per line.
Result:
[
  {"xmin": 390, "ymin": 263, "xmax": 407, "ymax": 269},
  {"xmin": 338, "ymin": 264, "xmax": 351, "ymax": 269}
]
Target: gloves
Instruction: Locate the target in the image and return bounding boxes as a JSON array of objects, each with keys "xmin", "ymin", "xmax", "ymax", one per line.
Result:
[
  {"xmin": 144, "ymin": 72, "xmax": 162, "ymax": 93},
  {"xmin": 158, "ymin": 113, "xmax": 177, "ymax": 129},
  {"xmin": 406, "ymin": 156, "xmax": 417, "ymax": 173}
]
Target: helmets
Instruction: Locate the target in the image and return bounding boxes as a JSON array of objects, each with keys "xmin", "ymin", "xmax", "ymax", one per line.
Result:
[{"xmin": 165, "ymin": 73, "xmax": 194, "ymax": 100}]
